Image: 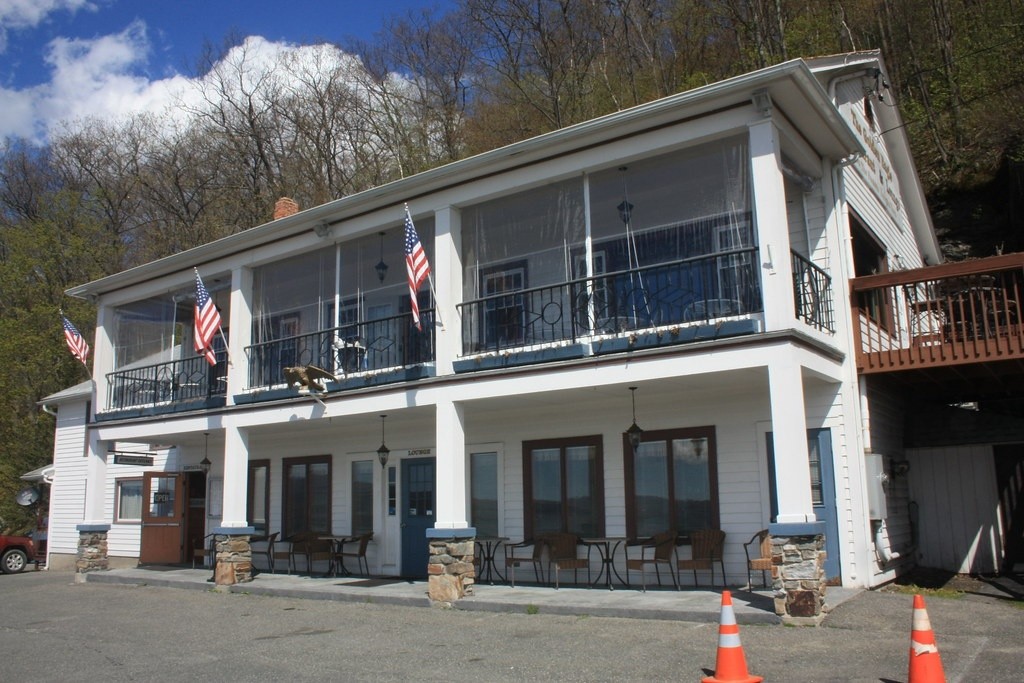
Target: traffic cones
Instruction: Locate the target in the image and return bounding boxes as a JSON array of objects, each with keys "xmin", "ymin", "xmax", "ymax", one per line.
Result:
[
  {"xmin": 908, "ymin": 594, "xmax": 946, "ymax": 683},
  {"xmin": 702, "ymin": 591, "xmax": 764, "ymax": 683}
]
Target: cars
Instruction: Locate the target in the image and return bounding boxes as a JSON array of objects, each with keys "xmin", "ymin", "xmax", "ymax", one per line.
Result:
[{"xmin": 0, "ymin": 535, "xmax": 36, "ymax": 574}]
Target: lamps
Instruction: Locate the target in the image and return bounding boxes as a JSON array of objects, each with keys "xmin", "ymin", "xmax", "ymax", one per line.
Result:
[
  {"xmin": 199, "ymin": 432, "xmax": 212, "ymax": 475},
  {"xmin": 627, "ymin": 387, "xmax": 644, "ymax": 454},
  {"xmin": 377, "ymin": 415, "xmax": 390, "ymax": 467},
  {"xmin": 374, "ymin": 233, "xmax": 389, "ymax": 280},
  {"xmin": 618, "ymin": 165, "xmax": 633, "ymax": 225}
]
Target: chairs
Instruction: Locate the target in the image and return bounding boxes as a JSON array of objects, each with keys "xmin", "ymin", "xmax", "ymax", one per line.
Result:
[
  {"xmin": 505, "ymin": 534, "xmax": 590, "ymax": 590},
  {"xmin": 192, "ymin": 532, "xmax": 372, "ymax": 578},
  {"xmin": 625, "ymin": 529, "xmax": 771, "ymax": 591},
  {"xmin": 910, "ymin": 299, "xmax": 946, "ymax": 347}
]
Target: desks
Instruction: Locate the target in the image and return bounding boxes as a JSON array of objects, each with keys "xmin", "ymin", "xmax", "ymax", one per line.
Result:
[
  {"xmin": 474, "ymin": 538, "xmax": 508, "ymax": 583},
  {"xmin": 318, "ymin": 537, "xmax": 358, "ymax": 578},
  {"xmin": 583, "ymin": 538, "xmax": 630, "ymax": 590}
]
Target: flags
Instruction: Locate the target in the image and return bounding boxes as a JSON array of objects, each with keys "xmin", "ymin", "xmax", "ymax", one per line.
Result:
[
  {"xmin": 63, "ymin": 319, "xmax": 88, "ymax": 365},
  {"xmin": 193, "ymin": 276, "xmax": 221, "ymax": 366},
  {"xmin": 405, "ymin": 211, "xmax": 430, "ymax": 330}
]
